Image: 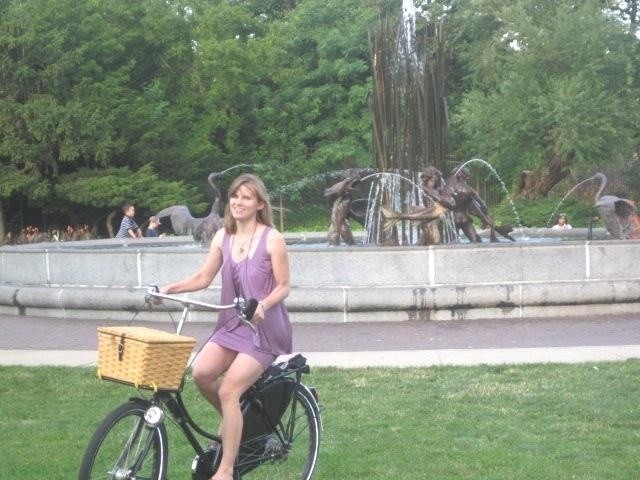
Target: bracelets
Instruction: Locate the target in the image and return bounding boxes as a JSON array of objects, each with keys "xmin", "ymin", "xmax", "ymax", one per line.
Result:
[{"xmin": 258, "ymin": 299, "xmax": 266, "ymax": 312}]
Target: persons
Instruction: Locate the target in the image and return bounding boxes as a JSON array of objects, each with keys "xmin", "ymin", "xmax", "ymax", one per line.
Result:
[
  {"xmin": 115, "ymin": 204, "xmax": 142, "ymax": 239},
  {"xmin": 0, "ymin": 223, "xmax": 89, "ymax": 244},
  {"xmin": 552, "ymin": 213, "xmax": 573, "ymax": 229},
  {"xmin": 144, "ymin": 215, "xmax": 164, "ymax": 238},
  {"xmin": 151, "ymin": 173, "xmax": 293, "ymax": 480}
]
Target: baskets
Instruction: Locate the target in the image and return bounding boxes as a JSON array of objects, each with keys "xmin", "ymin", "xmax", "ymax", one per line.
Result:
[{"xmin": 96, "ymin": 325, "xmax": 196, "ymax": 390}]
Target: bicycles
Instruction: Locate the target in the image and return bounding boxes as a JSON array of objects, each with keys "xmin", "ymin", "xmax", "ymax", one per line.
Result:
[{"xmin": 78, "ymin": 282, "xmax": 325, "ymax": 480}]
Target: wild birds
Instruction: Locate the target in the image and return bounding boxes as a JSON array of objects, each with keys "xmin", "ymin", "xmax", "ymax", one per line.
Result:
[
  {"xmin": 586, "ymin": 172, "xmax": 640, "ymax": 240},
  {"xmin": 150, "ymin": 172, "xmax": 224, "ymax": 246}
]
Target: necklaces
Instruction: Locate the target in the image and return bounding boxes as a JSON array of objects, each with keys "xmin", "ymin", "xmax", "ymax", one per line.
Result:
[{"xmin": 235, "ymin": 225, "xmax": 251, "ymax": 255}]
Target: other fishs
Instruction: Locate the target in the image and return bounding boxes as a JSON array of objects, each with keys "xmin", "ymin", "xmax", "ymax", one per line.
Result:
[{"xmin": 381, "ymin": 175, "xmax": 458, "ymax": 232}]
